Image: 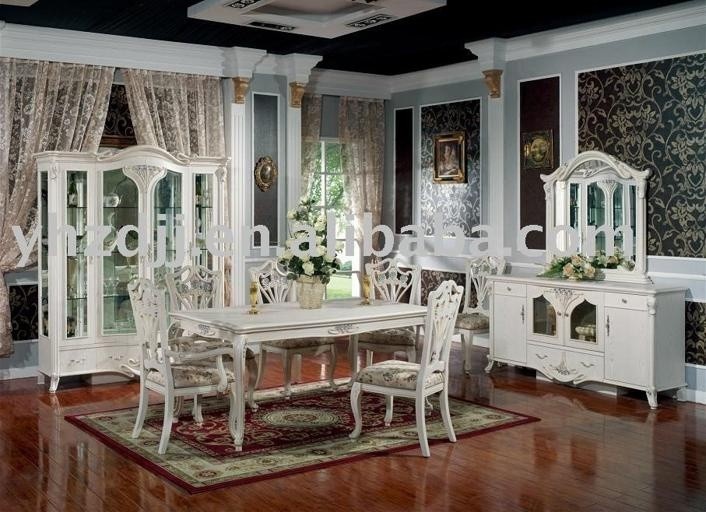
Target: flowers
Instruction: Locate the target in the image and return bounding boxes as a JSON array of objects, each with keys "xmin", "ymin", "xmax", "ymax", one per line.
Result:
[
  {"xmin": 588, "ymin": 255, "xmax": 618, "ymax": 269},
  {"xmin": 279, "ymin": 193, "xmax": 344, "ymax": 285},
  {"xmin": 536, "ymin": 254, "xmax": 606, "ymax": 279}
]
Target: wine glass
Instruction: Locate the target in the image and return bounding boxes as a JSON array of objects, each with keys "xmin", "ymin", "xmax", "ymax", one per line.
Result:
[
  {"xmin": 104, "ymin": 276, "xmax": 120, "ymax": 295},
  {"xmin": 203, "ymin": 190, "xmax": 209, "ymax": 207}
]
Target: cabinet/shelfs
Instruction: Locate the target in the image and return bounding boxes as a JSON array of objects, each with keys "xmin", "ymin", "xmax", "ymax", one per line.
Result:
[
  {"xmin": 32, "ymin": 144, "xmax": 231, "ymax": 394},
  {"xmin": 484, "ymin": 276, "xmax": 527, "ymax": 374},
  {"xmin": 527, "ymin": 281, "xmax": 605, "ymax": 386},
  {"xmin": 605, "ymin": 287, "xmax": 688, "ymax": 410}
]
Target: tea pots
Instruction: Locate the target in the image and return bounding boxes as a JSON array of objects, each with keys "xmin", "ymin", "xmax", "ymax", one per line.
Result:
[{"xmin": 103, "ymin": 192, "xmax": 124, "ymax": 207}]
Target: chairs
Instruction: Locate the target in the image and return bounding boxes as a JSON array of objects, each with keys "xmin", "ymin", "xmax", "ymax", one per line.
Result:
[
  {"xmin": 454, "ymin": 255, "xmax": 507, "ymax": 376},
  {"xmin": 248, "ymin": 260, "xmax": 339, "ymax": 402},
  {"xmin": 164, "ymin": 264, "xmax": 261, "ymax": 413},
  {"xmin": 348, "ymin": 279, "xmax": 464, "ymax": 458},
  {"xmin": 126, "ymin": 277, "xmax": 238, "ymax": 456},
  {"xmin": 358, "ymin": 258, "xmax": 434, "ymax": 410}
]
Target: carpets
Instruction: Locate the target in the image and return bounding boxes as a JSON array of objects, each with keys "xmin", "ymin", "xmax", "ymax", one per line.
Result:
[{"xmin": 64, "ymin": 376, "xmax": 541, "ymax": 495}]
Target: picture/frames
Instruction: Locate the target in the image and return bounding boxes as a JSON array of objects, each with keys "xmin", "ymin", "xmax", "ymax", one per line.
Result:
[{"xmin": 432, "ymin": 130, "xmax": 466, "ymax": 184}]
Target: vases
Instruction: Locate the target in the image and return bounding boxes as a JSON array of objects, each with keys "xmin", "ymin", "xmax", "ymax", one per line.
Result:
[{"xmin": 296, "ymin": 275, "xmax": 324, "ymax": 309}]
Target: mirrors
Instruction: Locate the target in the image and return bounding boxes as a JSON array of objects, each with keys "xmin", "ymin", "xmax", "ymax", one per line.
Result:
[{"xmin": 540, "ymin": 150, "xmax": 653, "ymax": 285}]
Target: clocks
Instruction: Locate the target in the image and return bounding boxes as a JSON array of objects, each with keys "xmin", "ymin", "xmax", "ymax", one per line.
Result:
[{"xmin": 520, "ymin": 128, "xmax": 554, "ymax": 177}]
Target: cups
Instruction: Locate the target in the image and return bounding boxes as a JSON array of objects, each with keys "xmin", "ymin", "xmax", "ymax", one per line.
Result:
[
  {"xmin": 248, "ymin": 281, "xmax": 260, "ymax": 314},
  {"xmin": 361, "ymin": 275, "xmax": 372, "ymax": 305}
]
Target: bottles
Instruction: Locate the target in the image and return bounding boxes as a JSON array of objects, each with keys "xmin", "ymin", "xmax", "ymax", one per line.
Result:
[
  {"xmin": 196, "ymin": 176, "xmax": 201, "ymax": 207},
  {"xmin": 69, "ymin": 174, "xmax": 77, "ymax": 208}
]
